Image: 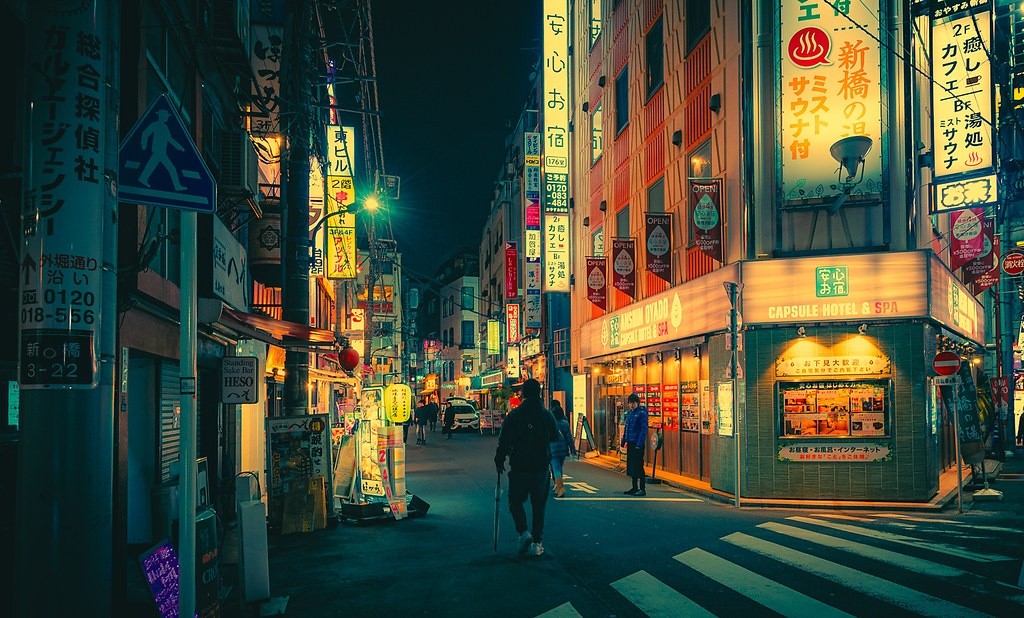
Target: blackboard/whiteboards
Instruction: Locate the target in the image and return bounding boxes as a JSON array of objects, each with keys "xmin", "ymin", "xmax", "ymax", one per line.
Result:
[
  {"xmin": 574, "ymin": 413, "xmax": 597, "ymax": 452},
  {"xmin": 138, "ymin": 538, "xmax": 179, "ymax": 618}
]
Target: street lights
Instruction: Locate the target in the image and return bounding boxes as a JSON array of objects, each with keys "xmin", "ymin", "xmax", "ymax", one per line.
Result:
[
  {"xmin": 363, "ymin": 345, "xmax": 393, "ymax": 383},
  {"xmin": 285, "ymin": 195, "xmax": 380, "ymax": 416}
]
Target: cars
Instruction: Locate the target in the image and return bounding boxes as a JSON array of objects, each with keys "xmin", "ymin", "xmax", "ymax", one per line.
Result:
[{"xmin": 441, "ymin": 397, "xmax": 480, "ymax": 432}]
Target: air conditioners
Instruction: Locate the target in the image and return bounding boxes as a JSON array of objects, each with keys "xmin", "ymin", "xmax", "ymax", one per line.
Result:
[{"xmin": 219, "ymin": 129, "xmax": 259, "ymax": 203}]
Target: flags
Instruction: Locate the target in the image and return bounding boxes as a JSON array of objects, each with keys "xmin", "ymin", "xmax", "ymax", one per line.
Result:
[
  {"xmin": 989, "ymin": 376, "xmax": 1009, "ymax": 451},
  {"xmin": 938, "ymin": 360, "xmax": 986, "ymax": 465}
]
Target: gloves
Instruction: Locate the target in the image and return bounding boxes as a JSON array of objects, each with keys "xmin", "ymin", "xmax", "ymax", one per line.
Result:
[
  {"xmin": 635, "ymin": 448, "xmax": 641, "ymax": 455},
  {"xmin": 621, "ymin": 440, "xmax": 625, "ymax": 447}
]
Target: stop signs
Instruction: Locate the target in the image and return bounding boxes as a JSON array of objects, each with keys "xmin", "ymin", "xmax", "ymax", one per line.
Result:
[{"xmin": 932, "ymin": 352, "xmax": 963, "ymax": 376}]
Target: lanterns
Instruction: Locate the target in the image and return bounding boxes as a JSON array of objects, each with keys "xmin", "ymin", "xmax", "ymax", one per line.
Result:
[
  {"xmin": 384, "ymin": 383, "xmax": 411, "ymax": 423},
  {"xmin": 339, "ymin": 348, "xmax": 359, "ymax": 371}
]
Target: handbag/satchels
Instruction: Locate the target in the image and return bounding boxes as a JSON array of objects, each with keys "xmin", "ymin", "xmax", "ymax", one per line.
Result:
[{"xmin": 442, "ymin": 426, "xmax": 448, "ymax": 434}]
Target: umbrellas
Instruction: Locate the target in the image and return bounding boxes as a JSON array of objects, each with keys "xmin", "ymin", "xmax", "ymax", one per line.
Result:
[{"xmin": 493, "ymin": 472, "xmax": 503, "ymax": 551}]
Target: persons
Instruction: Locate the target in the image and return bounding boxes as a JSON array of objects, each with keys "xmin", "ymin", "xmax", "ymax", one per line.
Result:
[
  {"xmin": 395, "ymin": 414, "xmax": 413, "ymax": 446},
  {"xmin": 494, "ymin": 378, "xmax": 559, "ymax": 556},
  {"xmin": 549, "ymin": 400, "xmax": 577, "ymax": 498},
  {"xmin": 621, "ymin": 394, "xmax": 648, "ymax": 496},
  {"xmin": 444, "ymin": 402, "xmax": 455, "ymax": 440},
  {"xmin": 1016, "ymin": 406, "xmax": 1024, "ymax": 450},
  {"xmin": 414, "ymin": 398, "xmax": 439, "ymax": 446}
]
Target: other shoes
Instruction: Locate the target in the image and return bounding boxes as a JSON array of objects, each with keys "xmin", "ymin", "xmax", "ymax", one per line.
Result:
[
  {"xmin": 530, "ymin": 542, "xmax": 545, "ymax": 555},
  {"xmin": 416, "ymin": 439, "xmax": 421, "ymax": 444},
  {"xmin": 633, "ymin": 489, "xmax": 646, "ymax": 496},
  {"xmin": 422, "ymin": 440, "xmax": 425, "ymax": 445},
  {"xmin": 518, "ymin": 530, "xmax": 533, "ymax": 554},
  {"xmin": 624, "ymin": 488, "xmax": 638, "ymax": 495}
]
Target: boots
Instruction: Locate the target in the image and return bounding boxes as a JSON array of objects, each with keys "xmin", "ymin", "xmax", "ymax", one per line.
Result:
[{"xmin": 555, "ymin": 478, "xmax": 566, "ymax": 498}]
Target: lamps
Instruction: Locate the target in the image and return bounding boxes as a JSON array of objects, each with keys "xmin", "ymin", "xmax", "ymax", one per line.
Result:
[
  {"xmin": 640, "ymin": 358, "xmax": 645, "ymax": 365},
  {"xmin": 826, "ymin": 136, "xmax": 873, "ymax": 216},
  {"xmin": 693, "ymin": 348, "xmax": 699, "ymax": 358},
  {"xmin": 858, "ymin": 324, "xmax": 868, "ymax": 335},
  {"xmin": 655, "ymin": 355, "xmax": 660, "ymax": 364},
  {"xmin": 797, "ymin": 326, "xmax": 806, "ymax": 338},
  {"xmin": 674, "ymin": 352, "xmax": 679, "ymax": 362},
  {"xmin": 625, "ymin": 361, "xmax": 631, "ymax": 368}
]
